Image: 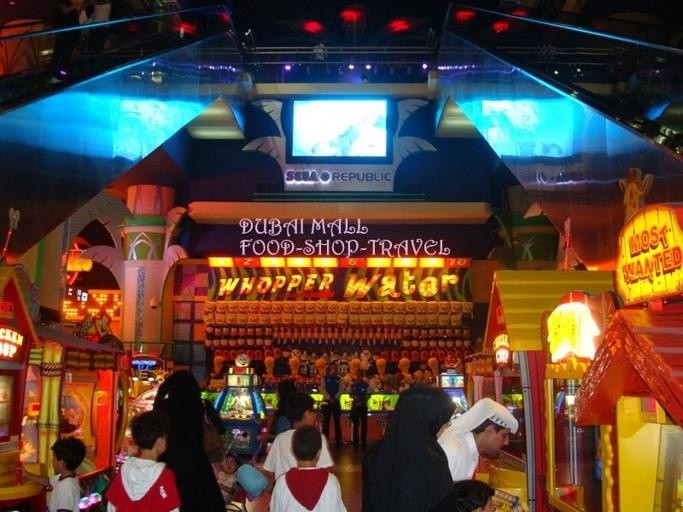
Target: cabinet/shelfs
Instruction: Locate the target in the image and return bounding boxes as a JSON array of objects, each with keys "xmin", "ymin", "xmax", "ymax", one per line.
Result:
[{"xmin": 200, "ymin": 391, "xmax": 400, "ymax": 445}]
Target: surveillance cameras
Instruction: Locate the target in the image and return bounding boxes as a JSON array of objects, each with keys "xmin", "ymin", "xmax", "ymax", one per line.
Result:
[{"xmin": 149, "ymin": 296, "xmax": 161, "ymax": 310}]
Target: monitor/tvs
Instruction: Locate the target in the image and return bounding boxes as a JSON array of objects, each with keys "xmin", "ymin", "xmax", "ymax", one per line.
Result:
[
  {"xmin": 285, "ymin": 95, "xmax": 394, "ymax": 165},
  {"xmin": 0, "ymin": 374, "xmax": 15, "ymax": 442}
]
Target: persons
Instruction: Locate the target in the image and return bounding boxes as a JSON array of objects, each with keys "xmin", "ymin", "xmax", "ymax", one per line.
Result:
[
  {"xmin": 361, "ymin": 385, "xmax": 457, "ymax": 512},
  {"xmin": 449, "ymin": 480, "xmax": 495, "ymax": 512},
  {"xmin": 16, "ymin": 435, "xmax": 87, "ymax": 512},
  {"xmin": 349, "ymin": 370, "xmax": 371, "ymax": 449},
  {"xmin": 104, "ymin": 410, "xmax": 182, "ymax": 512},
  {"xmin": 153, "ymin": 354, "xmax": 205, "ymax": 512},
  {"xmin": 216, "ymin": 452, "xmax": 240, "ymax": 505},
  {"xmin": 262, "ymin": 392, "xmax": 338, "ymax": 494},
  {"xmin": 269, "ymin": 425, "xmax": 348, "ymax": 512},
  {"xmin": 269, "ymin": 379, "xmax": 297, "ymax": 436},
  {"xmin": 436, "ymin": 397, "xmax": 518, "ymax": 483},
  {"xmin": 321, "ymin": 362, "xmax": 345, "ymax": 447},
  {"xmin": 201, "ymin": 399, "xmax": 228, "ymax": 481}
]
